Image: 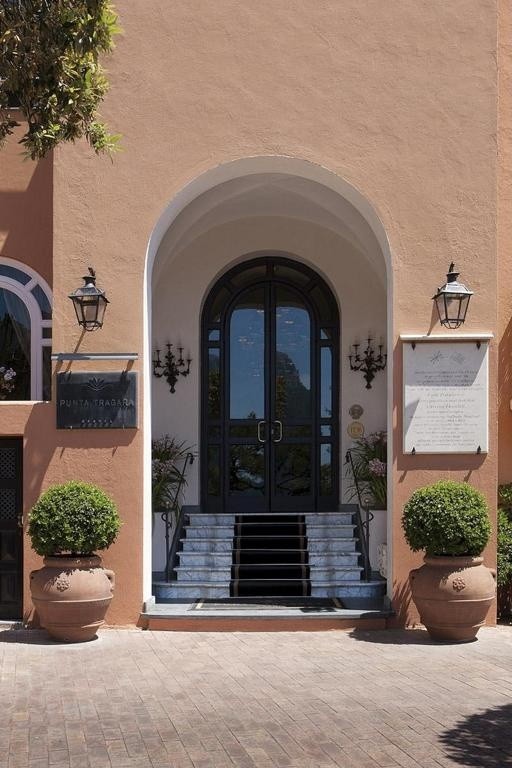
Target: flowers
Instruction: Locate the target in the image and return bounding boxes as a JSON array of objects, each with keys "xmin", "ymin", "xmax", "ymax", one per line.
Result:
[
  {"xmin": 151, "ymin": 432, "xmax": 200, "ymax": 524},
  {"xmin": 343, "ymin": 430, "xmax": 387, "ymax": 505}
]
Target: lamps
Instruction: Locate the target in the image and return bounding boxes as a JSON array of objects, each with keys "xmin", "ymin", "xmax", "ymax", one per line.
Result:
[
  {"xmin": 152, "ymin": 344, "xmax": 192, "ymax": 393},
  {"xmin": 348, "ymin": 338, "xmax": 387, "ymax": 389},
  {"xmin": 431, "ymin": 262, "xmax": 474, "ymax": 329},
  {"xmin": 67, "ymin": 266, "xmax": 110, "ymax": 332}
]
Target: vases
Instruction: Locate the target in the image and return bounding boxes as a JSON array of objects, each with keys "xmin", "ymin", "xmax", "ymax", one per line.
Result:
[
  {"xmin": 357, "ymin": 481, "xmax": 385, "ymax": 506},
  {"xmin": 160, "ymin": 482, "xmax": 179, "ymax": 508}
]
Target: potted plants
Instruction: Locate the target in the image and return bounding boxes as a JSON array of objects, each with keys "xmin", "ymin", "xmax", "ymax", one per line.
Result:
[
  {"xmin": 26, "ymin": 481, "xmax": 123, "ymax": 644},
  {"xmin": 400, "ymin": 480, "xmax": 497, "ymax": 643}
]
